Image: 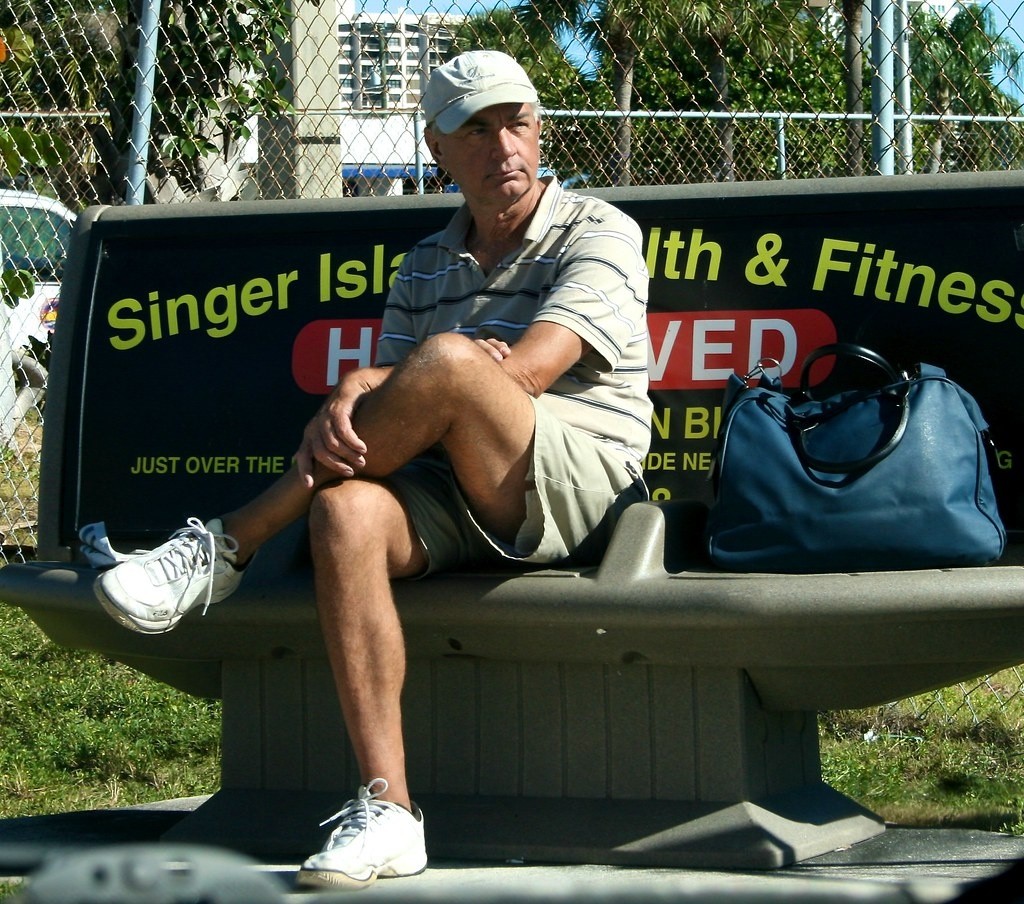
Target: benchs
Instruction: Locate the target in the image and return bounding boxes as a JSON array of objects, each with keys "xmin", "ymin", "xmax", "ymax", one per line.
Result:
[{"xmin": 0, "ymin": 169, "xmax": 1024, "ymax": 866}]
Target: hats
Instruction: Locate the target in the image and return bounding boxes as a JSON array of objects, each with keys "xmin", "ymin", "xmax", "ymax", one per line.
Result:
[{"xmin": 422, "ymin": 51, "xmax": 540, "ymax": 134}]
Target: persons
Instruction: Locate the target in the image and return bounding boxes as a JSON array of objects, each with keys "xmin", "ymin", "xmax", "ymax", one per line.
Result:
[{"xmin": 97, "ymin": 50, "xmax": 654, "ymax": 891}]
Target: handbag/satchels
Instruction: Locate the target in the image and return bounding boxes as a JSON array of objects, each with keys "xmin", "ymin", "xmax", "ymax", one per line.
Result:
[{"xmin": 704, "ymin": 343, "xmax": 1007, "ymax": 573}]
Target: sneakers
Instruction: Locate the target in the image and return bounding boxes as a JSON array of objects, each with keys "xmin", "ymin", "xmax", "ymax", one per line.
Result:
[
  {"xmin": 92, "ymin": 516, "xmax": 260, "ymax": 634},
  {"xmin": 294, "ymin": 778, "xmax": 428, "ymax": 890}
]
